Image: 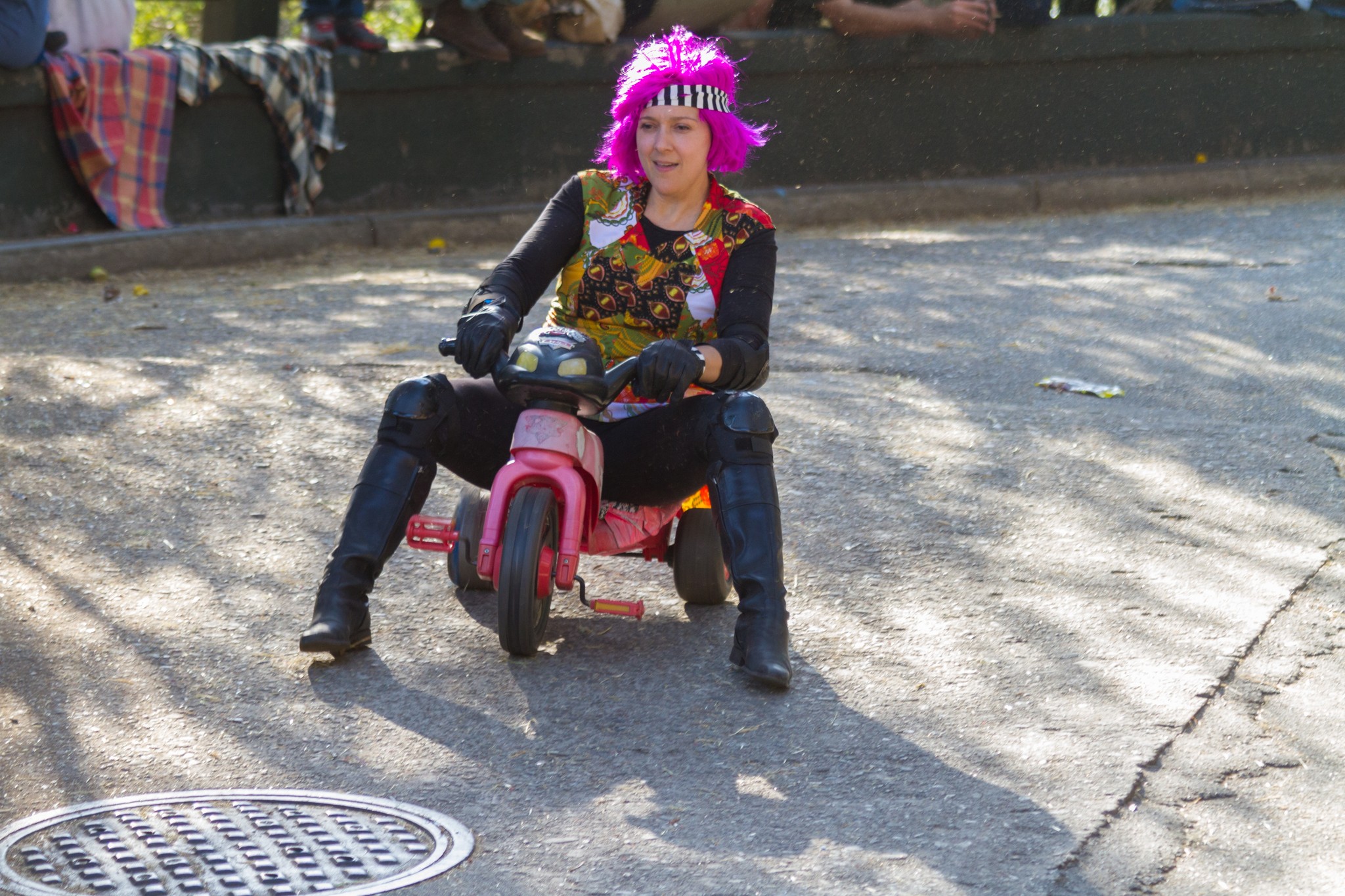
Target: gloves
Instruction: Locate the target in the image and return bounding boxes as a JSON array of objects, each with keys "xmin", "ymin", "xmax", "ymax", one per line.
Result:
[
  {"xmin": 455, "ymin": 295, "xmax": 518, "ymax": 380},
  {"xmin": 630, "ymin": 338, "xmax": 703, "ymax": 413}
]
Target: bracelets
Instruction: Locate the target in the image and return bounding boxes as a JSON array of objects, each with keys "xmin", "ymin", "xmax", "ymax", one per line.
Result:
[{"xmin": 690, "ymin": 347, "xmax": 706, "ymax": 374}]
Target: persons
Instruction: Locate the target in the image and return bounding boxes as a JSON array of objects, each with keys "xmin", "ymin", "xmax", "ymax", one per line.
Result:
[
  {"xmin": 1, "ymin": 0, "xmax": 1344, "ymax": 75},
  {"xmin": 299, "ymin": 27, "xmax": 795, "ymax": 684}
]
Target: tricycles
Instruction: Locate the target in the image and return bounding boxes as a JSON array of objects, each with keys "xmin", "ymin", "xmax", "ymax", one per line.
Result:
[{"xmin": 402, "ymin": 326, "xmax": 732, "ymax": 653}]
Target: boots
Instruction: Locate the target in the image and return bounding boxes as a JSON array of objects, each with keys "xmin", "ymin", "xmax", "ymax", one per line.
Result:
[
  {"xmin": 703, "ymin": 451, "xmax": 792, "ymax": 687},
  {"xmin": 300, "ymin": 444, "xmax": 439, "ymax": 654}
]
